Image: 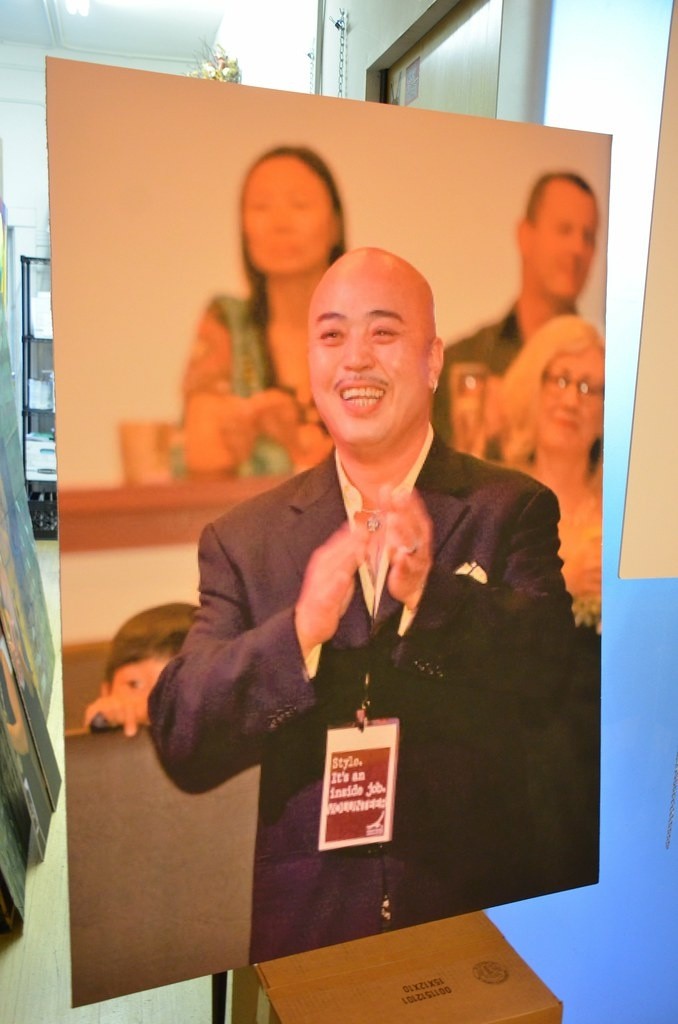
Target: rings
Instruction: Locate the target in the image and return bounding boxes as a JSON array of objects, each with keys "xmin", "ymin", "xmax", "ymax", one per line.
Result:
[{"xmin": 408, "ymin": 547, "xmax": 415, "ymax": 557}]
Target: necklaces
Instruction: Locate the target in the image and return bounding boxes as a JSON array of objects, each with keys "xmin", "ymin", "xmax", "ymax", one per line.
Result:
[{"xmin": 359, "ymin": 508, "xmax": 387, "ymax": 532}]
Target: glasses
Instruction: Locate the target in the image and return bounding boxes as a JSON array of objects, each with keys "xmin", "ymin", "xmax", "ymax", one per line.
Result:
[{"xmin": 546, "ymin": 374, "xmax": 603, "ymax": 396}]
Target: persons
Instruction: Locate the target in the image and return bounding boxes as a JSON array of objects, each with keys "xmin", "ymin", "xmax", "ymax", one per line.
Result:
[{"xmin": 84, "ymin": 145, "xmax": 605, "ymax": 963}]
